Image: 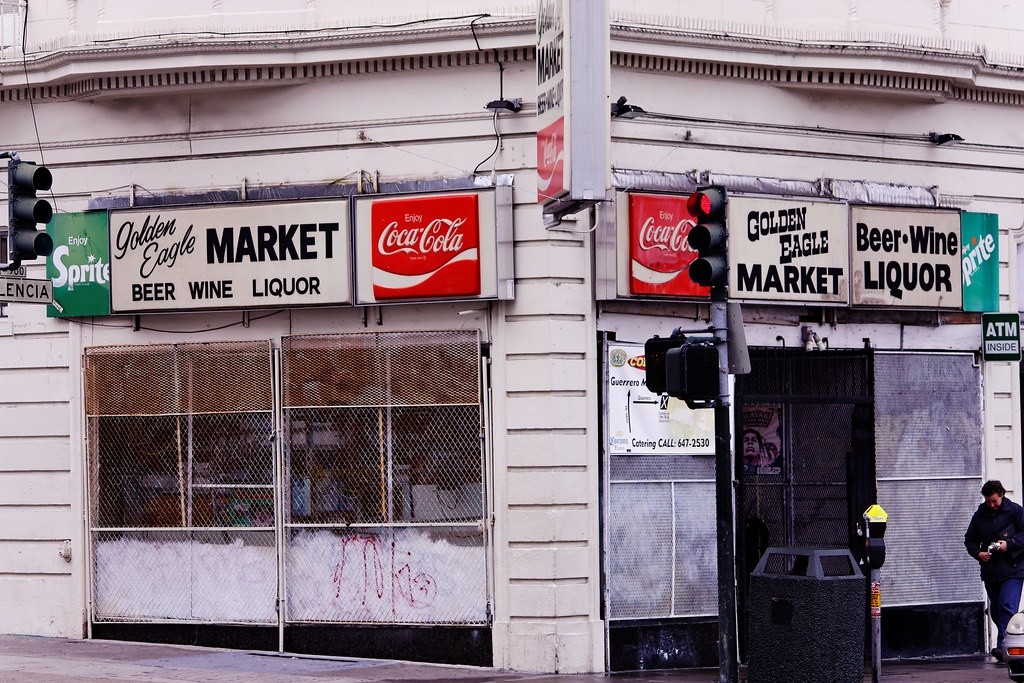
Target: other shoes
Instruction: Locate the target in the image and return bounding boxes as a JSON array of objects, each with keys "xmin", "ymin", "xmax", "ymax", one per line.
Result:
[
  {"xmin": 991, "ymin": 648, "xmax": 1005, "ymax": 662},
  {"xmin": 995, "ymin": 661, "xmax": 1008, "ymax": 668}
]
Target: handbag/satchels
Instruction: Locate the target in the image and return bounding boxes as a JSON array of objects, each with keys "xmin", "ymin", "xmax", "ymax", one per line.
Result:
[{"xmin": 997, "ymin": 521, "xmax": 1024, "ymax": 567}]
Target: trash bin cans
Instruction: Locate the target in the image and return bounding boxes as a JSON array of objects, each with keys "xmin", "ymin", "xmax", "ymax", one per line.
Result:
[{"xmin": 748, "ymin": 546, "xmax": 868, "ymax": 682}]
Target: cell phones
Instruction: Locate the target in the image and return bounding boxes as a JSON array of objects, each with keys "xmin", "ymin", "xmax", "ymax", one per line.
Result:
[{"xmin": 990, "ymin": 542, "xmax": 1000, "ymax": 550}]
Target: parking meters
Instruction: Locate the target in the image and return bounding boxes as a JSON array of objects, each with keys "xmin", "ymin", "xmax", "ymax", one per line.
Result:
[{"xmin": 863, "ymin": 505, "xmax": 887, "ymax": 683}]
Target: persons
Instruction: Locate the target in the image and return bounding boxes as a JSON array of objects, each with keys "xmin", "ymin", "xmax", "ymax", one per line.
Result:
[{"xmin": 962, "ymin": 480, "xmax": 1023, "ymax": 664}]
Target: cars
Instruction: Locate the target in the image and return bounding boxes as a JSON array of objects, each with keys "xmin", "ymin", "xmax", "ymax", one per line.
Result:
[{"xmin": 1002, "ymin": 610, "xmax": 1024, "ymax": 682}]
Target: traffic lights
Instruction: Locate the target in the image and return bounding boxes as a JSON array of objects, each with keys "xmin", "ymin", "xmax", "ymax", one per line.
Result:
[
  {"xmin": 644, "ymin": 326, "xmax": 722, "ymax": 409},
  {"xmin": 7, "ymin": 159, "xmax": 53, "ymax": 260},
  {"xmin": 685, "ymin": 185, "xmax": 728, "ymax": 285}
]
